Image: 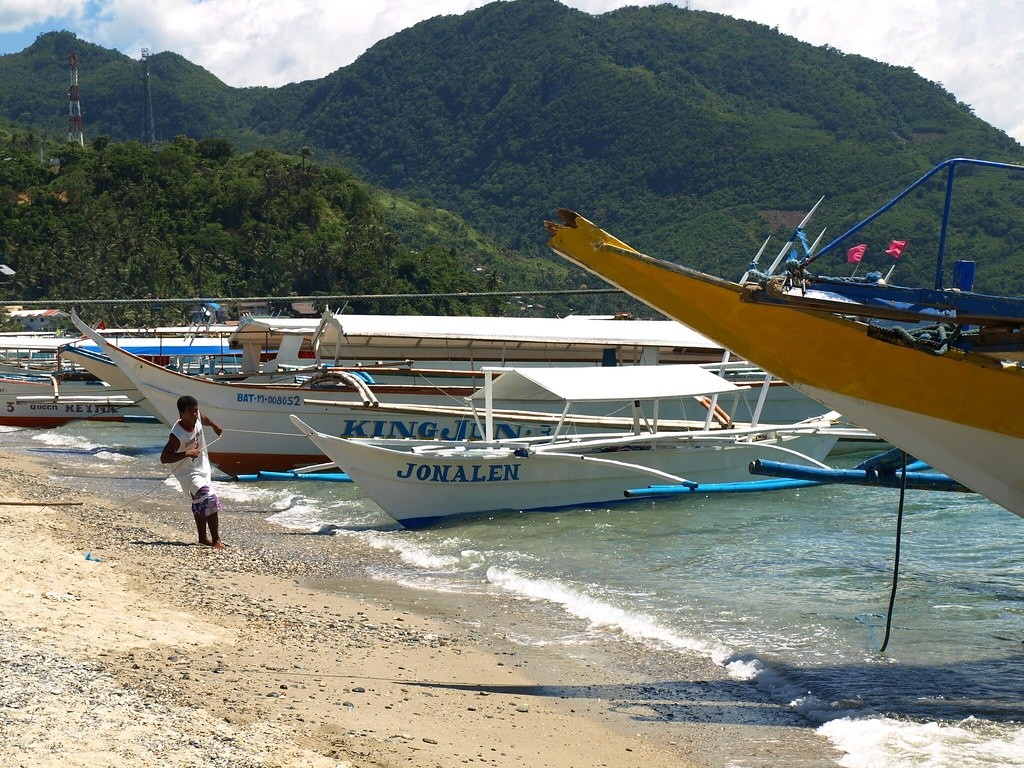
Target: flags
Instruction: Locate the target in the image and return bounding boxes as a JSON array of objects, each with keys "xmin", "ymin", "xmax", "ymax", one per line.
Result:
[
  {"xmin": 885, "ymin": 240, "xmax": 906, "ymax": 258},
  {"xmin": 847, "ymin": 244, "xmax": 866, "ymax": 262},
  {"xmin": 98, "ymin": 321, "xmax": 106, "ymax": 330}
]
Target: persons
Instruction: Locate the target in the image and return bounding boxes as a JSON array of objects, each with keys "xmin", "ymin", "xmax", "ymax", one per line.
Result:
[{"xmin": 161, "ymin": 396, "xmax": 228, "ymax": 548}]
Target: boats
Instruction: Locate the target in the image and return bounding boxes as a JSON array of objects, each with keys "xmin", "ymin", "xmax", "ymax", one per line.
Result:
[
  {"xmin": 1, "ymin": 284, "xmax": 763, "ymax": 479},
  {"xmin": 543, "ymin": 154, "xmax": 1023, "ymax": 518},
  {"xmin": 286, "ymin": 363, "xmax": 845, "ymax": 533}
]
